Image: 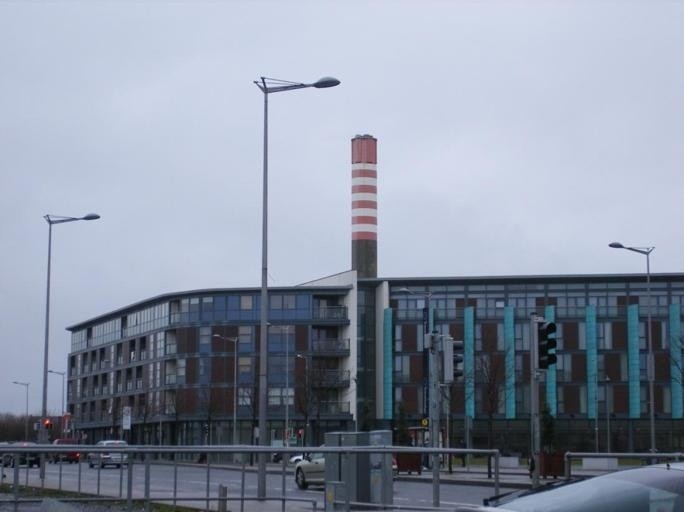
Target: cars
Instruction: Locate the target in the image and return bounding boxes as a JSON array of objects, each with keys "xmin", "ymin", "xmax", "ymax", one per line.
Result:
[
  {"xmin": 295, "ymin": 442, "xmax": 400, "ymax": 490},
  {"xmin": 0, "ymin": 439, "xmax": 130, "ymax": 468},
  {"xmin": 486, "ymin": 459, "xmax": 684, "ymax": 512}
]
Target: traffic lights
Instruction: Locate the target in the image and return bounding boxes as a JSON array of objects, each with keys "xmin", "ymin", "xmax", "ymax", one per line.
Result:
[
  {"xmin": 528, "ymin": 315, "xmax": 558, "ymax": 372},
  {"xmin": 442, "ymin": 335, "xmax": 464, "ymax": 385}
]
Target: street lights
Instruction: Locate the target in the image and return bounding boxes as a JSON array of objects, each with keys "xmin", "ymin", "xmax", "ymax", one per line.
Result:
[
  {"xmin": 212, "ymin": 334, "xmax": 239, "ymax": 448},
  {"xmin": 39, "ymin": 212, "xmax": 101, "ymax": 445},
  {"xmin": 253, "ymin": 75, "xmax": 341, "ymax": 499},
  {"xmin": 12, "ymin": 381, "xmax": 29, "ymax": 440},
  {"xmin": 48, "ymin": 369, "xmax": 65, "ymax": 415},
  {"xmin": 607, "ymin": 241, "xmax": 657, "ymax": 464}
]
